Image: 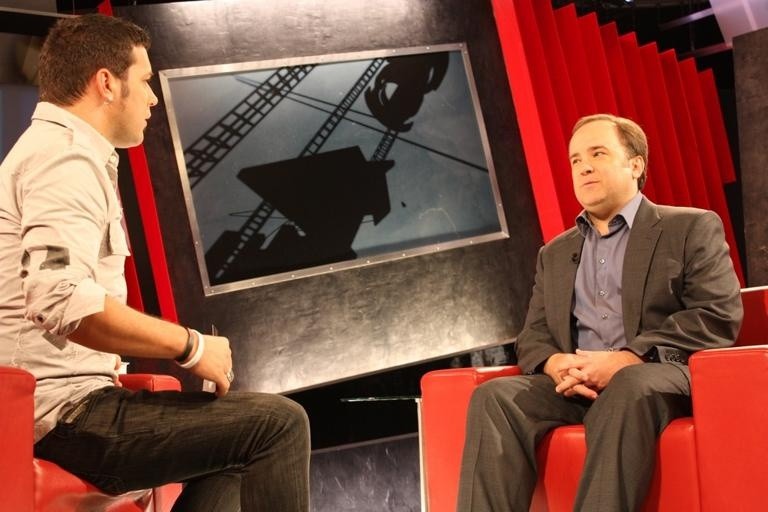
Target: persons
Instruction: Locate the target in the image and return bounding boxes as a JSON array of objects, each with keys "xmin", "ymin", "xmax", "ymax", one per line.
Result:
[
  {"xmin": 0, "ymin": 12, "xmax": 315, "ymax": 511},
  {"xmin": 453, "ymin": 113, "xmax": 747, "ymax": 512}
]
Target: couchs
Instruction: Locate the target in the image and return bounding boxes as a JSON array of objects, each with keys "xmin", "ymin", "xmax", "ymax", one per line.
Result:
[
  {"xmin": 417, "ymin": 286, "xmax": 768, "ymax": 511},
  {"xmin": 2, "ymin": 357, "xmax": 190, "ymax": 512}
]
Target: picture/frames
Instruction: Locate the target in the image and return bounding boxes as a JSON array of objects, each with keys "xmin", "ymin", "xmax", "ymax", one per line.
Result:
[{"xmin": 158, "ymin": 42, "xmax": 511, "ymax": 298}]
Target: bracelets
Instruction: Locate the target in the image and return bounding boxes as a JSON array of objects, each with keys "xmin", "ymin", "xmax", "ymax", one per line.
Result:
[
  {"xmin": 173, "ymin": 328, "xmax": 205, "ymax": 371},
  {"xmin": 177, "ymin": 326, "xmax": 194, "ymax": 361}
]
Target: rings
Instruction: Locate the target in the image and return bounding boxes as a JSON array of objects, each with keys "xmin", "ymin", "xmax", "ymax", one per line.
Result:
[{"xmin": 227, "ymin": 368, "xmax": 234, "ymax": 379}]
